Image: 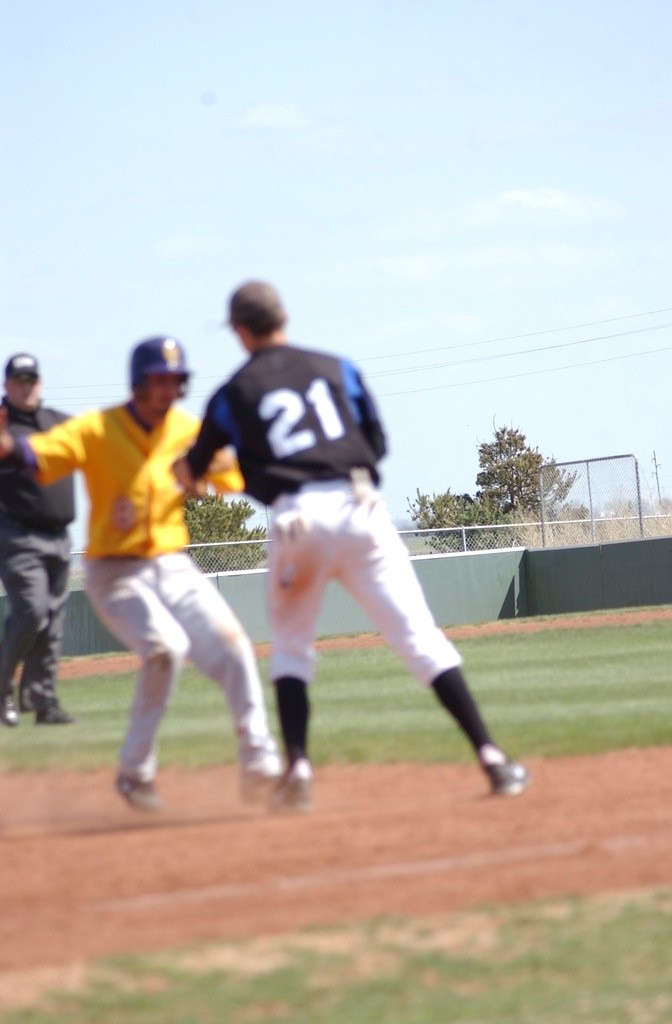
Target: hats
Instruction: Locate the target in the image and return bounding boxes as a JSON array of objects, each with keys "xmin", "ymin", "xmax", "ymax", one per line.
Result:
[
  {"xmin": 224, "ymin": 282, "xmax": 281, "ymax": 321},
  {"xmin": 6, "ymin": 353, "xmax": 39, "ymax": 378}
]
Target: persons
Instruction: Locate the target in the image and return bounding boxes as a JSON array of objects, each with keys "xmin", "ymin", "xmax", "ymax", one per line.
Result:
[
  {"xmin": 1, "ymin": 332, "xmax": 289, "ymax": 811},
  {"xmin": 182, "ymin": 282, "xmax": 527, "ymax": 798},
  {"xmin": 2, "ymin": 351, "xmax": 88, "ymax": 727}
]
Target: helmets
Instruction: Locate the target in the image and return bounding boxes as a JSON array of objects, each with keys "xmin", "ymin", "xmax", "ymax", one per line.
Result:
[{"xmin": 131, "ymin": 338, "xmax": 191, "ymax": 383}]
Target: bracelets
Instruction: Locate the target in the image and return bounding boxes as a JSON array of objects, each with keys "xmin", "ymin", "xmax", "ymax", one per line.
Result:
[{"xmin": 206, "ymin": 481, "xmax": 216, "ymax": 498}]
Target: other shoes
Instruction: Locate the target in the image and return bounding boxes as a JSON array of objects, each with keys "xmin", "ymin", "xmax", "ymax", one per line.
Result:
[
  {"xmin": 36, "ymin": 708, "xmax": 74, "ymax": 722},
  {"xmin": 0, "ymin": 694, "xmax": 19, "ymax": 726},
  {"xmin": 19, "ymin": 696, "xmax": 33, "ymax": 712}
]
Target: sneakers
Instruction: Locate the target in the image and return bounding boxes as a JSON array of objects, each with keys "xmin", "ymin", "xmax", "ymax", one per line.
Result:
[
  {"xmin": 115, "ymin": 776, "xmax": 164, "ymax": 811},
  {"xmin": 269, "ymin": 779, "xmax": 311, "ymax": 808},
  {"xmin": 240, "ymin": 767, "xmax": 284, "ymax": 795},
  {"xmin": 481, "ymin": 754, "xmax": 528, "ymax": 797}
]
